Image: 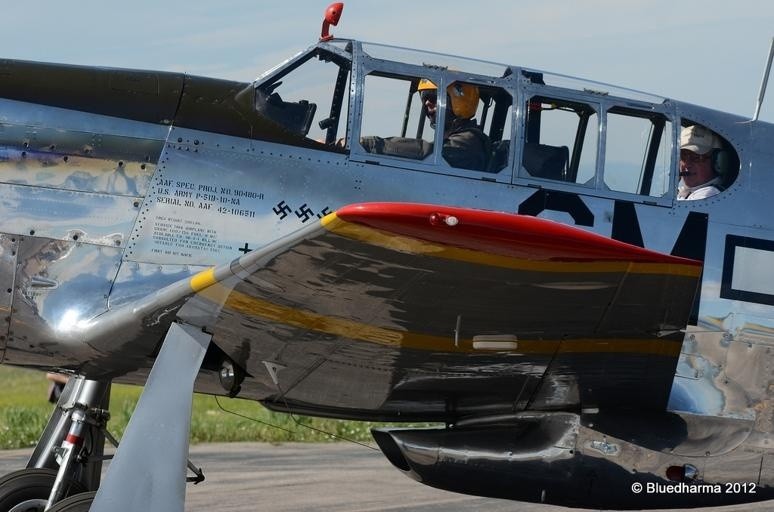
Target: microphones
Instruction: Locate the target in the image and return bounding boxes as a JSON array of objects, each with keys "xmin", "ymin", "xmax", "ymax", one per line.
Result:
[{"xmin": 680, "ymin": 169, "xmax": 692, "ymax": 177}]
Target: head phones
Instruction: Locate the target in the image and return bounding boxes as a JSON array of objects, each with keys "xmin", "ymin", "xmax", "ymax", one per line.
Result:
[{"xmin": 712, "ymin": 142, "xmax": 730, "ymax": 176}]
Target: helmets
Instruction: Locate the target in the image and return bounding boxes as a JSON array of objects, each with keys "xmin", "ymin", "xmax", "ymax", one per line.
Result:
[
  {"xmin": 679, "ymin": 125, "xmax": 732, "ymax": 175},
  {"xmin": 417, "ymin": 78, "xmax": 480, "ymax": 120}
]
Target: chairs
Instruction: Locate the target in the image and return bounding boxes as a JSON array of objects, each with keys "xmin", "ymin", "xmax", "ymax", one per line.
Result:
[{"xmin": 485, "ymin": 138, "xmax": 568, "ymax": 181}]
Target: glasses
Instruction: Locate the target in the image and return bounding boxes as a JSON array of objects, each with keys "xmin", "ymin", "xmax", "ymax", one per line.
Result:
[{"xmin": 680, "ymin": 149, "xmax": 715, "ymax": 164}]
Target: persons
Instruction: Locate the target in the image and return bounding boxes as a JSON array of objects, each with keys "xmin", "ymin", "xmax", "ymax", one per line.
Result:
[
  {"xmin": 676, "ymin": 125, "xmax": 730, "ymax": 201},
  {"xmin": 315, "ymin": 77, "xmax": 496, "ymax": 174}
]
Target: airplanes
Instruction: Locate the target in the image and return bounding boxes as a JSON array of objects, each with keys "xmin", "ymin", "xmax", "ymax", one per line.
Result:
[{"xmin": 0, "ymin": 4, "xmax": 773, "ymax": 510}]
